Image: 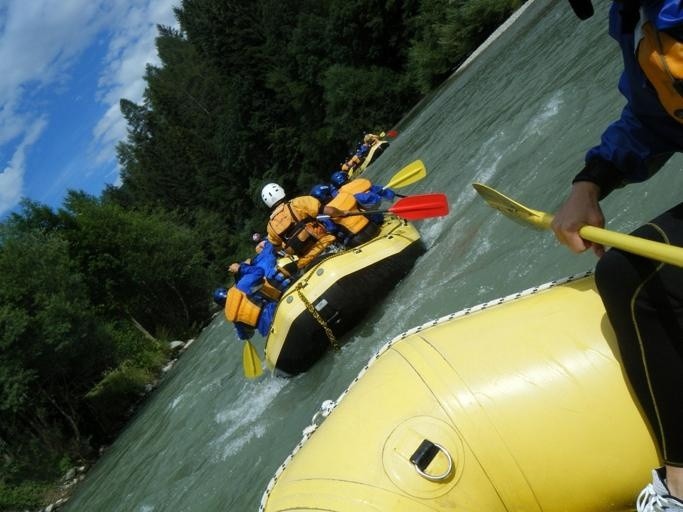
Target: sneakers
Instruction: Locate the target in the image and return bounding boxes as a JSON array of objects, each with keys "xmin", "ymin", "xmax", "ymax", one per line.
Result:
[{"xmin": 636, "ymin": 466, "xmax": 683, "ymax": 512}]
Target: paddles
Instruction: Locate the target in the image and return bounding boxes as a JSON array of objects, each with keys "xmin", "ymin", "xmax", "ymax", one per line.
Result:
[
  {"xmin": 472, "ymin": 183, "xmax": 683, "ymax": 268},
  {"xmin": 382, "ymin": 159, "xmax": 427, "ymax": 190},
  {"xmin": 378, "ymin": 131, "xmax": 386, "ymax": 139},
  {"xmin": 316, "ymin": 193, "xmax": 448, "ymax": 220},
  {"xmin": 234, "ymin": 272, "xmax": 263, "ymax": 378}
]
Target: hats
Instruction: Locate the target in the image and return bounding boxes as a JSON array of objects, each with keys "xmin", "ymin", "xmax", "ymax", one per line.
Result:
[{"xmin": 261, "ymin": 183, "xmax": 286, "ymax": 208}]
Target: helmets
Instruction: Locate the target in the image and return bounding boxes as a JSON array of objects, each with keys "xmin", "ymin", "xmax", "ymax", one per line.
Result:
[
  {"xmin": 214, "ymin": 288, "xmax": 226, "ymax": 305},
  {"xmin": 252, "ymin": 233, "xmax": 260, "ymax": 242},
  {"xmin": 331, "ymin": 171, "xmax": 347, "ymax": 185},
  {"xmin": 309, "ymin": 183, "xmax": 330, "ymax": 201}
]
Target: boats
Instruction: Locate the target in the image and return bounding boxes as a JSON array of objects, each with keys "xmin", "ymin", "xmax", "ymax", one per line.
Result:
[
  {"xmin": 264, "ymin": 213, "xmax": 428, "ymax": 378},
  {"xmin": 347, "ymin": 140, "xmax": 390, "ymax": 180}
]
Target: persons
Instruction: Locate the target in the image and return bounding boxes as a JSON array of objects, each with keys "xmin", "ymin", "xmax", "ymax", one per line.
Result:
[
  {"xmin": 204, "ymin": 171, "xmax": 394, "ymax": 343},
  {"xmin": 548, "ymin": 0, "xmax": 683, "ymax": 512},
  {"xmin": 334, "ymin": 131, "xmax": 381, "ymax": 170}
]
